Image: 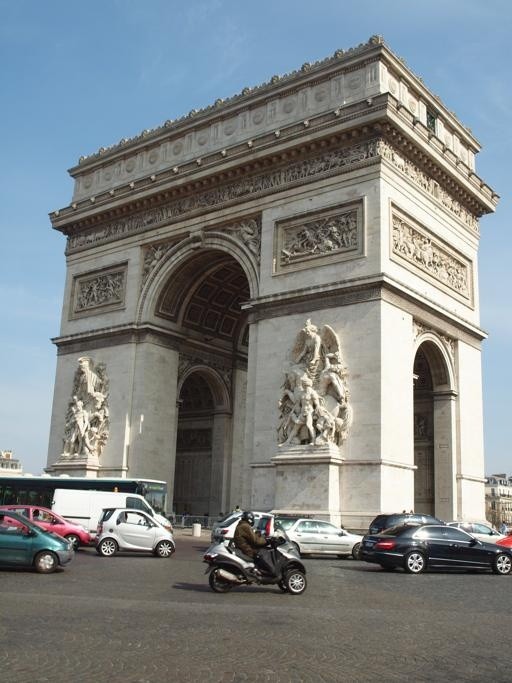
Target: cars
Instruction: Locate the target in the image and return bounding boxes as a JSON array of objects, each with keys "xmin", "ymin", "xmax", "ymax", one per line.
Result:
[
  {"xmin": 96, "ymin": 507, "xmax": 176, "ymax": 557},
  {"xmin": 0, "ymin": 502, "xmax": 92, "ymax": 574}
]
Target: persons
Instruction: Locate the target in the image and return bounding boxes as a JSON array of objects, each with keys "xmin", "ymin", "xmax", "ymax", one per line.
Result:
[
  {"xmin": 273, "ymin": 316, "xmax": 353, "ymax": 449},
  {"xmin": 68, "ymin": 136, "xmax": 380, "ymax": 251},
  {"xmin": 381, "ymin": 137, "xmax": 479, "ymax": 233},
  {"xmin": 499, "ymin": 521, "xmax": 508, "ymax": 535},
  {"xmin": 74, "ymin": 273, "xmax": 121, "ymax": 311},
  {"xmin": 58, "ymin": 354, "xmax": 109, "ymax": 458},
  {"xmin": 282, "ymin": 214, "xmax": 358, "ymax": 264},
  {"xmin": 392, "ymin": 216, "xmax": 467, "ymax": 294},
  {"xmin": 33, "ymin": 509, "xmax": 40, "ymax": 521},
  {"xmin": 235, "ymin": 509, "xmax": 285, "ymax": 584}
]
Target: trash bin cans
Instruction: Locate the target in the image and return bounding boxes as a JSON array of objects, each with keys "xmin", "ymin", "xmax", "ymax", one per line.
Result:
[{"xmin": 193, "ymin": 524, "xmax": 201, "ymax": 537}]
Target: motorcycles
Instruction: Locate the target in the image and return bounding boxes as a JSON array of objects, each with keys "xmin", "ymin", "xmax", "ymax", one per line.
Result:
[{"xmin": 198, "ymin": 522, "xmax": 307, "ymax": 594}]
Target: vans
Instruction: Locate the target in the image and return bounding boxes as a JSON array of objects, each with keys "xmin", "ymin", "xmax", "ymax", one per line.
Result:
[{"xmin": 49, "ymin": 487, "xmax": 173, "ymax": 543}]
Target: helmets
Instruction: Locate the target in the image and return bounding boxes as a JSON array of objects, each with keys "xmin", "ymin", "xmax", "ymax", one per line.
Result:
[{"xmin": 242, "ymin": 511, "xmax": 255, "ymax": 526}]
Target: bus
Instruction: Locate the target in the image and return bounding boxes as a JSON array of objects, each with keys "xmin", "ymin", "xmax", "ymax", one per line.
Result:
[{"xmin": 0, "ymin": 475, "xmax": 170, "ymax": 521}]
